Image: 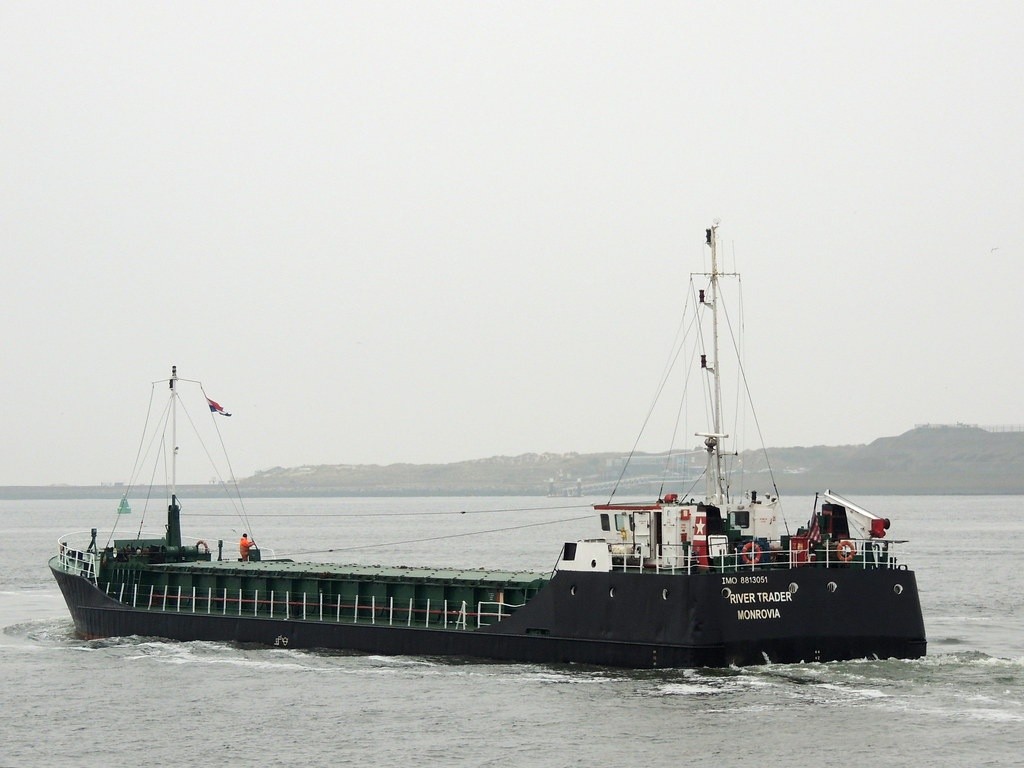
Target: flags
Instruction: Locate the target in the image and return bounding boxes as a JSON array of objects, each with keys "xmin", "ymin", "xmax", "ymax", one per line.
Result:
[{"xmin": 207, "ymin": 398, "xmax": 232, "ymax": 417}]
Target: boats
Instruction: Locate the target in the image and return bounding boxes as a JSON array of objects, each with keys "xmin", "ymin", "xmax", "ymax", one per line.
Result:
[{"xmin": 44, "ymin": 212, "xmax": 925, "ymax": 678}]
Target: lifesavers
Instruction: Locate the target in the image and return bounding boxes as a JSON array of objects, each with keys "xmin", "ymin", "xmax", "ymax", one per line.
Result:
[
  {"xmin": 195, "ymin": 541, "xmax": 209, "ymax": 555},
  {"xmin": 741, "ymin": 542, "xmax": 761, "ymax": 564},
  {"xmin": 836, "ymin": 541, "xmax": 855, "ymax": 561}
]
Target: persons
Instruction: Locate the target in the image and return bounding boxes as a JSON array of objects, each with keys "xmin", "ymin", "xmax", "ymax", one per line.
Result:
[{"xmin": 238, "ymin": 533, "xmax": 255, "ymax": 562}]
